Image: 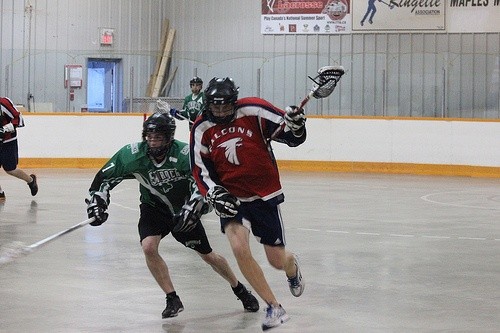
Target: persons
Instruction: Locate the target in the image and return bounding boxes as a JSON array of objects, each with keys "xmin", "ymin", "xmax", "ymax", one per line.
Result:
[
  {"xmin": 188, "ymin": 77, "xmax": 308, "ymax": 331},
  {"xmin": 83, "ymin": 113, "xmax": 259, "ymax": 319},
  {"xmin": 170, "ymin": 77, "xmax": 206, "ymax": 130},
  {"xmin": 0, "ymin": 97, "xmax": 38, "ymax": 199}
]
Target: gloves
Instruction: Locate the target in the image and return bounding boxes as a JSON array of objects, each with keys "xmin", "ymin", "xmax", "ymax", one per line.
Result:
[
  {"xmin": 283, "ymin": 105, "xmax": 308, "ymax": 138},
  {"xmin": 171, "ymin": 199, "xmax": 208, "ymax": 233},
  {"xmin": 170, "ymin": 108, "xmax": 180, "ymax": 118},
  {"xmin": 204, "ymin": 185, "xmax": 241, "ymax": 219},
  {"xmin": 0, "ymin": 123, "xmax": 15, "ymax": 134},
  {"xmin": 84, "ymin": 194, "xmax": 109, "ymax": 226}
]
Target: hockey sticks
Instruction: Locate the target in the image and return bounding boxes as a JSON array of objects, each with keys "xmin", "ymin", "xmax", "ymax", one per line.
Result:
[
  {"xmin": 156, "ymin": 98, "xmax": 194, "ymax": 124},
  {"xmin": 0, "ymin": 215, "xmax": 95, "ymax": 272},
  {"xmin": 267, "ymin": 66, "xmax": 347, "ymax": 144}
]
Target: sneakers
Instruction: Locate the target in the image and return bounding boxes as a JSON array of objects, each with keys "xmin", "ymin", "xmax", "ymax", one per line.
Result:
[
  {"xmin": 0, "ymin": 190, "xmax": 6, "ymax": 199},
  {"xmin": 27, "ymin": 174, "xmax": 38, "ymax": 196},
  {"xmin": 262, "ymin": 304, "xmax": 290, "ymax": 330},
  {"xmin": 161, "ymin": 296, "xmax": 184, "ymax": 317},
  {"xmin": 231, "ymin": 281, "xmax": 260, "ymax": 312},
  {"xmin": 286, "ymin": 253, "xmax": 305, "ymax": 297}
]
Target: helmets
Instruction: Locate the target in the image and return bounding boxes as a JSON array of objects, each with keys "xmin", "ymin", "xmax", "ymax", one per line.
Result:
[
  {"xmin": 205, "ymin": 77, "xmax": 241, "ymax": 126},
  {"xmin": 189, "ymin": 77, "xmax": 203, "ymax": 93},
  {"xmin": 142, "ymin": 113, "xmax": 176, "ymax": 155}
]
